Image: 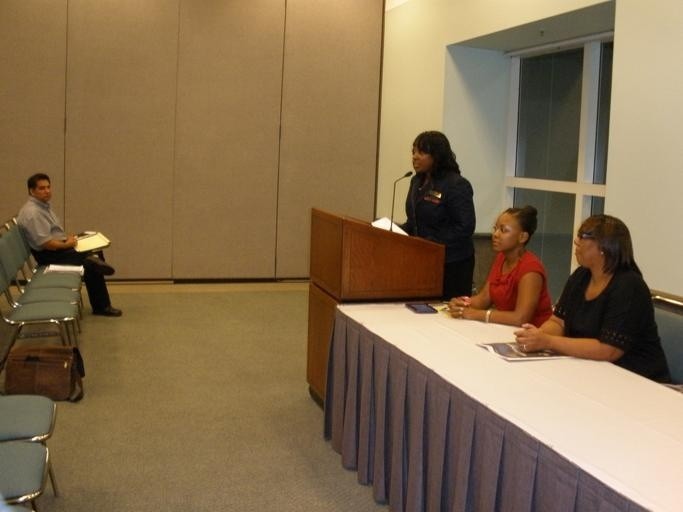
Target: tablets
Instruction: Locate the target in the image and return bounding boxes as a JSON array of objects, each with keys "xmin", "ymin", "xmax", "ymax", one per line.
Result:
[{"xmin": 406, "ymin": 302, "xmax": 438, "ymax": 314}]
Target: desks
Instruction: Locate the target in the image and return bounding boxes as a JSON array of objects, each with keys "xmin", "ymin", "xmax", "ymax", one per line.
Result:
[{"xmin": 324, "ymin": 301, "xmax": 683, "ymax": 510}]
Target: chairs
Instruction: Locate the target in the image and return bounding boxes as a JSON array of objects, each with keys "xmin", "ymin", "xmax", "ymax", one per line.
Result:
[
  {"xmin": 644, "ymin": 294, "xmax": 682, "ymax": 391},
  {"xmin": 0, "ymin": 215, "xmax": 85, "ymax": 509}
]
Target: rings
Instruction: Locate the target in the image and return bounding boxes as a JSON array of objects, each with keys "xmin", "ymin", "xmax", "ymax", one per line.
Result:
[{"xmin": 522, "ymin": 345, "xmax": 526, "ymax": 351}]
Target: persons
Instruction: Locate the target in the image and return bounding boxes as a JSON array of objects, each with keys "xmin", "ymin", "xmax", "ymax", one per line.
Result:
[
  {"xmin": 374, "ymin": 130, "xmax": 475, "ymax": 301},
  {"xmin": 16, "ymin": 173, "xmax": 123, "ymax": 317},
  {"xmin": 447, "ymin": 204, "xmax": 553, "ymax": 328},
  {"xmin": 512, "ymin": 214, "xmax": 671, "ymax": 385}
]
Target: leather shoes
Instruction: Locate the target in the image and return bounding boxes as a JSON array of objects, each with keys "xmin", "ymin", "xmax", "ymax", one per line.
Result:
[
  {"xmin": 83, "ymin": 256, "xmax": 114, "ymax": 275},
  {"xmin": 92, "ymin": 305, "xmax": 122, "ymax": 316}
]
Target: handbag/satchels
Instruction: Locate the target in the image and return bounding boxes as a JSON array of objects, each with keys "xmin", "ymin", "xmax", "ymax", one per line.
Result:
[{"xmin": 5, "ymin": 346, "xmax": 85, "ymax": 402}]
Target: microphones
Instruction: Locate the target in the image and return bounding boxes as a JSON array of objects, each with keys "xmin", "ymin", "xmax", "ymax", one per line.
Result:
[{"xmin": 389, "ymin": 170, "xmax": 413, "ymax": 233}]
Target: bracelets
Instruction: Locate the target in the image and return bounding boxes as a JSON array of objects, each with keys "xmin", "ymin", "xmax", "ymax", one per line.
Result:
[{"xmin": 484, "ymin": 309, "xmax": 492, "ymax": 323}]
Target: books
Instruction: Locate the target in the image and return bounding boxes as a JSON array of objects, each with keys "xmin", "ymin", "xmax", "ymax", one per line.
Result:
[
  {"xmin": 43, "ymin": 263, "xmax": 84, "ymax": 275},
  {"xmin": 72, "ymin": 231, "xmax": 111, "ymax": 253},
  {"xmin": 370, "ymin": 217, "xmax": 408, "ymax": 237}
]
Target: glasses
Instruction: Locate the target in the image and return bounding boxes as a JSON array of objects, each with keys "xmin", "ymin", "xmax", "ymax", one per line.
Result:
[{"xmin": 577, "ymin": 232, "xmax": 593, "ymax": 239}]
